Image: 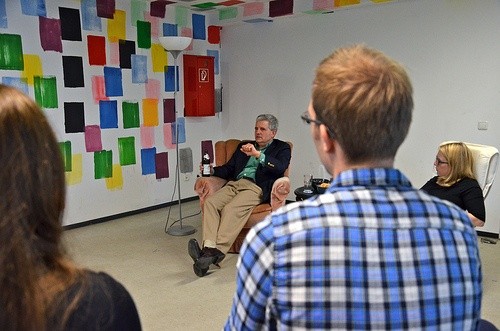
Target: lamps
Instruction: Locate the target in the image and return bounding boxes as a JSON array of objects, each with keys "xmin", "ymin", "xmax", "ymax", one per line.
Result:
[{"xmin": 159, "ymin": 36, "xmax": 197, "ymax": 236}]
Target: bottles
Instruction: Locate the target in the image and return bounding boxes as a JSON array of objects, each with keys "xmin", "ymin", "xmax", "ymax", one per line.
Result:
[{"xmin": 202, "ymin": 150, "xmax": 210, "ymax": 177}]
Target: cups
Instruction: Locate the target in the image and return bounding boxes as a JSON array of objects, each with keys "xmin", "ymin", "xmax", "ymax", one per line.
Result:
[{"xmin": 304, "ymin": 174, "xmax": 312, "ymax": 192}]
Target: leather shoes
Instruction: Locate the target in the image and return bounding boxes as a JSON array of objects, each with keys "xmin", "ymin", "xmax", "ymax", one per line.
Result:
[
  {"xmin": 195, "ymin": 247, "xmax": 226, "ymax": 269},
  {"xmin": 186, "ymin": 239, "xmax": 210, "ymax": 277}
]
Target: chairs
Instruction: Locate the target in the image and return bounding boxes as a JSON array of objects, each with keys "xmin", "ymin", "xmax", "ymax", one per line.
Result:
[{"xmin": 430, "ymin": 141, "xmax": 499, "ymax": 201}]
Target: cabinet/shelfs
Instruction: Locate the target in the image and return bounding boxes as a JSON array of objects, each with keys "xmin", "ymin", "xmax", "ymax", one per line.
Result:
[{"xmin": 183, "ymin": 53, "xmax": 216, "ymax": 117}]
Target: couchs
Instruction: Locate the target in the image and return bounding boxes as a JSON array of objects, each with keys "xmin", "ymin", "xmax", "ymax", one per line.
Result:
[{"xmin": 194, "ymin": 139, "xmax": 293, "ymax": 254}]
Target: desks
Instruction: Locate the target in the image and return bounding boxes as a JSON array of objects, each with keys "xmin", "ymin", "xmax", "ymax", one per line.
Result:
[{"xmin": 294, "ymin": 186, "xmax": 319, "ymax": 201}]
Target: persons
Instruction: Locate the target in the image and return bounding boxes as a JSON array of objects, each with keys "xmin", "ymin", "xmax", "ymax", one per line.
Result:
[
  {"xmin": 223, "ymin": 45, "xmax": 483, "ymax": 331},
  {"xmin": 188, "ymin": 114, "xmax": 291, "ymax": 276},
  {"xmin": 420, "ymin": 143, "xmax": 486, "ymax": 227},
  {"xmin": 0, "ymin": 84, "xmax": 143, "ymax": 331}
]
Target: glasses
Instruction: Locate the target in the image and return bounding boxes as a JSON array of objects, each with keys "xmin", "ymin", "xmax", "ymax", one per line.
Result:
[
  {"xmin": 301, "ymin": 112, "xmax": 336, "ymax": 140},
  {"xmin": 436, "ymin": 157, "xmax": 448, "ymax": 166}
]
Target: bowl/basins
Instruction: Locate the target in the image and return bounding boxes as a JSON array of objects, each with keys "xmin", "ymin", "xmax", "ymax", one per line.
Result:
[{"xmin": 312, "ymin": 179, "xmax": 328, "ymax": 189}]
